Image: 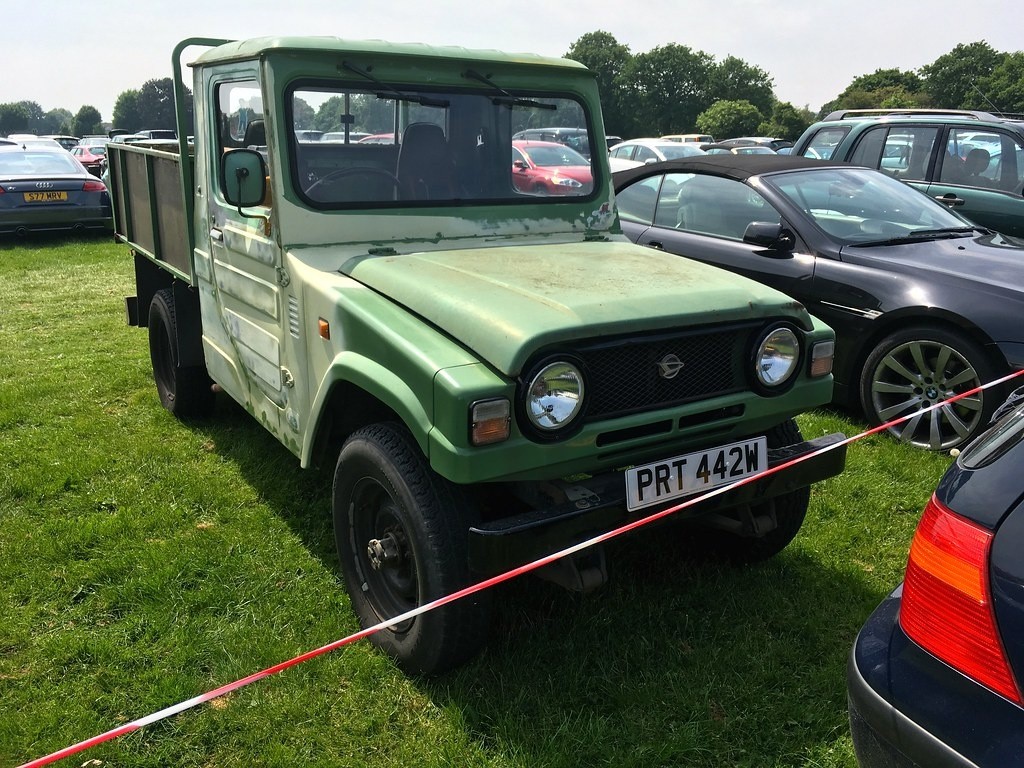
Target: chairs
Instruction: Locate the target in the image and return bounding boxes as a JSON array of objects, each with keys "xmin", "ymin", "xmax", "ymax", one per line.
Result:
[
  {"xmin": 922, "ymin": 150, "xmax": 961, "ymax": 184},
  {"xmin": 396, "ymin": 123, "xmax": 458, "ymax": 199},
  {"xmin": 964, "ymin": 148, "xmax": 994, "ymax": 187},
  {"xmin": 676, "ymin": 199, "xmax": 738, "ymax": 239},
  {"xmin": 244, "ymin": 118, "xmax": 324, "ymax": 202},
  {"xmin": 760, "ymin": 201, "xmax": 783, "ymax": 224}
]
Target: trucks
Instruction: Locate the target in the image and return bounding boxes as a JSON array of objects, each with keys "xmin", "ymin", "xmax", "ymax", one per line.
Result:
[{"xmin": 102, "ymin": 35, "xmax": 852, "ymax": 680}]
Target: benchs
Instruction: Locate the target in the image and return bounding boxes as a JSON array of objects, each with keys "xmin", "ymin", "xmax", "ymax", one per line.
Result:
[{"xmin": 629, "ymin": 185, "xmax": 760, "ymax": 230}]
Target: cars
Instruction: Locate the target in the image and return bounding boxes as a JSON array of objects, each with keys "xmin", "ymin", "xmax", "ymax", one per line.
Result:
[
  {"xmin": 506, "ymin": 128, "xmax": 1022, "ymax": 204},
  {"xmin": 568, "ymin": 153, "xmax": 1024, "ymax": 462},
  {"xmin": 0, "ymin": 128, "xmax": 406, "ymax": 190},
  {"xmin": 0, "ymin": 143, "xmax": 116, "ymax": 237},
  {"xmin": 847, "ymin": 388, "xmax": 1024, "ymax": 768}
]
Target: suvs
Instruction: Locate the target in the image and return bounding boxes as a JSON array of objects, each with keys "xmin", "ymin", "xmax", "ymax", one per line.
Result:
[{"xmin": 787, "ymin": 108, "xmax": 1024, "ymax": 240}]
